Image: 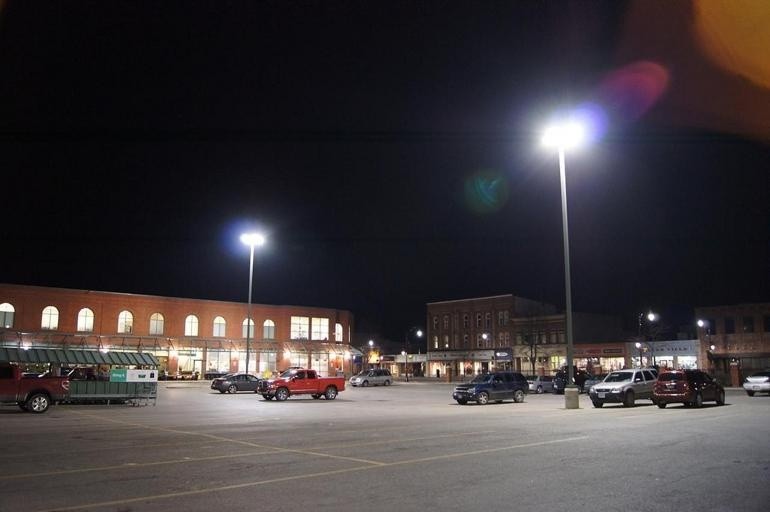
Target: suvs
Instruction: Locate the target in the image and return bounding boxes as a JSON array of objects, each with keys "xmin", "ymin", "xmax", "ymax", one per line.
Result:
[
  {"xmin": 452, "ymin": 371, "xmax": 529, "ymax": 405},
  {"xmin": 589, "ymin": 368, "xmax": 658, "ymax": 408}
]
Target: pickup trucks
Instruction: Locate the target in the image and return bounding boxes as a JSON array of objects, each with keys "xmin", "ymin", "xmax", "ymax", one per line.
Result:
[
  {"xmin": 257, "ymin": 368, "xmax": 345, "ymax": 401},
  {"xmin": 0, "ymin": 361, "xmax": 70, "ymax": 413},
  {"xmin": 38, "ymin": 366, "xmax": 127, "ymax": 405}
]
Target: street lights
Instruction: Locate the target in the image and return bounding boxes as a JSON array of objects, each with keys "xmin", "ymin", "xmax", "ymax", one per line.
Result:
[
  {"xmin": 240, "ymin": 231, "xmax": 265, "ymax": 374},
  {"xmin": 697, "ymin": 319, "xmax": 716, "ymax": 379},
  {"xmin": 400, "ymin": 327, "xmax": 422, "ymax": 382},
  {"xmin": 482, "ymin": 332, "xmax": 500, "ymax": 371},
  {"xmin": 539, "ymin": 117, "xmax": 588, "ymax": 409},
  {"xmin": 634, "ymin": 311, "xmax": 654, "ymax": 368},
  {"xmin": 369, "ymin": 340, "xmax": 384, "ymax": 369}
]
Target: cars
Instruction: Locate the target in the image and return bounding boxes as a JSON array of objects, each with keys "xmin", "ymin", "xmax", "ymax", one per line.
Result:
[
  {"xmin": 348, "ymin": 369, "xmax": 393, "ymax": 387},
  {"xmin": 525, "ymin": 374, "xmax": 555, "ymax": 393},
  {"xmin": 743, "ymin": 371, "xmax": 770, "ymax": 398},
  {"xmin": 584, "ymin": 372, "xmax": 610, "ymax": 395},
  {"xmin": 211, "ymin": 372, "xmax": 268, "ymax": 393},
  {"xmin": 653, "ymin": 369, "xmax": 725, "ymax": 408},
  {"xmin": 553, "ymin": 370, "xmax": 594, "ymax": 394}
]
